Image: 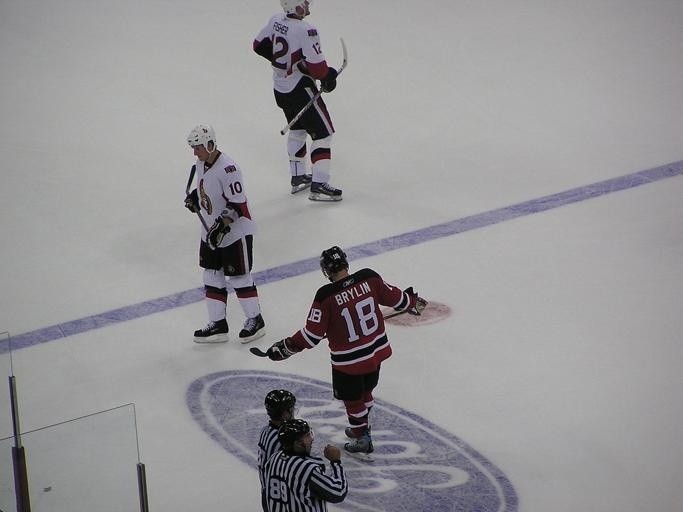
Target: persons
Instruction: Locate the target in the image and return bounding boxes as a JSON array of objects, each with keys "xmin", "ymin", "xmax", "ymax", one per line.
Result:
[
  {"xmin": 178, "ymin": 123, "xmax": 264, "ymax": 338},
  {"xmin": 258, "ymin": 389, "xmax": 297, "ymax": 512},
  {"xmin": 253, "ymin": 0, "xmax": 342, "ymax": 197},
  {"xmin": 265, "ymin": 420, "xmax": 348, "ymax": 512},
  {"xmin": 266, "ymin": 247, "xmax": 417, "ymax": 453}
]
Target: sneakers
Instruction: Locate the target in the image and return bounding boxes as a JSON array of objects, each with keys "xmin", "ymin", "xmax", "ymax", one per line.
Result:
[
  {"xmin": 194, "ymin": 318, "xmax": 229, "ymax": 339},
  {"xmin": 345, "ymin": 425, "xmax": 370, "ymax": 439},
  {"xmin": 309, "ymin": 178, "xmax": 343, "ymax": 198},
  {"xmin": 342, "ymin": 434, "xmax": 374, "ymax": 453},
  {"xmin": 238, "ymin": 313, "xmax": 265, "ymax": 337},
  {"xmin": 289, "ymin": 171, "xmax": 312, "ymax": 187}
]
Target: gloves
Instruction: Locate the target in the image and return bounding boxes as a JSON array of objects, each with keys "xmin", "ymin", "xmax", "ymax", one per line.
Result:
[
  {"xmin": 395, "ymin": 287, "xmax": 419, "ymax": 315},
  {"xmin": 268, "ymin": 338, "xmax": 294, "ymax": 361},
  {"xmin": 206, "ymin": 216, "xmax": 231, "ymax": 252},
  {"xmin": 320, "ymin": 62, "xmax": 338, "ymax": 93},
  {"xmin": 183, "ymin": 189, "xmax": 202, "ymax": 213}
]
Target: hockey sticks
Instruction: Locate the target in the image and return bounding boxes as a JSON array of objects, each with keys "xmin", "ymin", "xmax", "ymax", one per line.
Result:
[
  {"xmin": 250, "ymin": 310, "xmax": 407, "ymax": 357},
  {"xmin": 186, "ymin": 164, "xmax": 209, "ymax": 231},
  {"xmin": 280, "ymin": 38, "xmax": 347, "ymax": 135}
]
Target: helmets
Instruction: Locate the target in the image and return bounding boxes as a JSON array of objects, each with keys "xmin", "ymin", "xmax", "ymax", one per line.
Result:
[
  {"xmin": 185, "ymin": 123, "xmax": 215, "ymax": 153},
  {"xmin": 281, "ymin": 1, "xmax": 312, "ymax": 20},
  {"xmin": 318, "ymin": 245, "xmax": 349, "ymax": 278},
  {"xmin": 277, "ymin": 417, "xmax": 309, "ymax": 447},
  {"xmin": 264, "ymin": 388, "xmax": 296, "ymax": 412}
]
